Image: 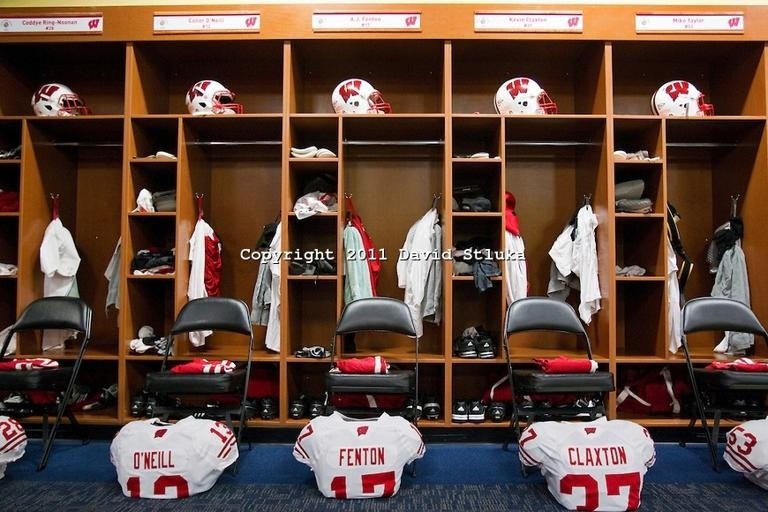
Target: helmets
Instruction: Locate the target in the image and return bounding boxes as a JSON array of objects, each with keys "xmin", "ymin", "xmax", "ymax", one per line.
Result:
[
  {"xmin": 30, "ymin": 83, "xmax": 89, "ymax": 116},
  {"xmin": 651, "ymin": 80, "xmax": 715, "ymax": 115},
  {"xmin": 184, "ymin": 80, "xmax": 244, "ymax": 115},
  {"xmin": 493, "ymin": 77, "xmax": 557, "ymax": 114},
  {"xmin": 331, "ymin": 78, "xmax": 392, "ymax": 114}
]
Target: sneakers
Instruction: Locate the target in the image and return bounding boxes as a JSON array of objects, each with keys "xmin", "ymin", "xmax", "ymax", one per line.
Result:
[
  {"xmin": 0, "ymin": 392, "xmax": 32, "ymax": 415},
  {"xmin": 474, "ymin": 335, "xmax": 495, "ymax": 359},
  {"xmin": 147, "ymin": 398, "xmax": 157, "ymax": 417},
  {"xmin": 309, "ymin": 399, "xmax": 324, "ymax": 418},
  {"xmin": 261, "ymin": 397, "xmax": 276, "ymax": 419},
  {"xmin": 57, "ymin": 385, "xmax": 88, "ymax": 407},
  {"xmin": 455, "ymin": 335, "xmax": 478, "ymax": 358},
  {"xmin": 452, "ymin": 397, "xmax": 604, "ymax": 423},
  {"xmin": 131, "ymin": 399, "xmax": 144, "ymax": 416},
  {"xmin": 83, "ymin": 385, "xmax": 118, "ymax": 411},
  {"xmin": 423, "ymin": 398, "xmax": 441, "ymax": 420},
  {"xmin": 407, "ymin": 399, "xmax": 422, "ymax": 420},
  {"xmin": 290, "ymin": 400, "xmax": 305, "ymax": 418},
  {"xmin": 239, "ymin": 397, "xmax": 258, "ymax": 420},
  {"xmin": 129, "ymin": 338, "xmax": 154, "ymax": 354},
  {"xmin": 153, "ymin": 338, "xmax": 171, "ymax": 355}
]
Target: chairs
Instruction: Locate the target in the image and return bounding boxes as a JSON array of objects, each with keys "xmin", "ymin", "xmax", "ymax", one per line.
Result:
[
  {"xmin": 139, "ymin": 296, "xmax": 255, "ymax": 477},
  {"xmin": 677, "ymin": 297, "xmax": 767, "ymax": 472},
  {"xmin": 1, "ymin": 297, "xmax": 96, "ymax": 473},
  {"xmin": 500, "ymin": 297, "xmax": 618, "ymax": 475},
  {"xmin": 324, "ymin": 297, "xmax": 422, "ymax": 477}
]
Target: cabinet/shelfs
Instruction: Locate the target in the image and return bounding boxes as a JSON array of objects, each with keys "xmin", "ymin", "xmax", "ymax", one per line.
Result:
[{"xmin": 0, "ymin": 6, "xmax": 767, "ymax": 429}]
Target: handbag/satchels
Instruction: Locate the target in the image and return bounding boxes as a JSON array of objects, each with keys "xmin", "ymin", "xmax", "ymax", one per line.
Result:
[
  {"xmin": 712, "ymin": 216, "xmax": 743, "ymax": 253},
  {"xmin": 616, "ymin": 366, "xmax": 689, "ymax": 419}
]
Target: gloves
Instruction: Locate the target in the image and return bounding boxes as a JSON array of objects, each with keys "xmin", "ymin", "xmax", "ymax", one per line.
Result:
[
  {"xmin": 303, "ymin": 346, "xmax": 325, "ymax": 357},
  {"xmin": 294, "ymin": 351, "xmax": 331, "ymax": 358}
]
